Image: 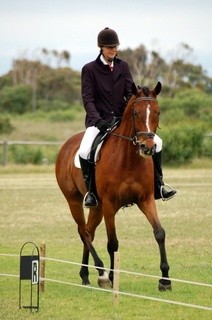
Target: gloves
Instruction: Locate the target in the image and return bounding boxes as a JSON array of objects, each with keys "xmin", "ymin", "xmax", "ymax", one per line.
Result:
[{"xmin": 96, "ymin": 119, "xmax": 112, "ymax": 133}]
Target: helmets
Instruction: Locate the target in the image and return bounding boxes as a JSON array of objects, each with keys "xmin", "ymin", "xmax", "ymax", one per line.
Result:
[{"xmin": 97, "ymin": 27, "xmax": 120, "ymax": 47}]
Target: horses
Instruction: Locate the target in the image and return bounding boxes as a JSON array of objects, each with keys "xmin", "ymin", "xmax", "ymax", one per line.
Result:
[{"xmin": 55, "ymin": 82, "xmax": 173, "ymax": 292}]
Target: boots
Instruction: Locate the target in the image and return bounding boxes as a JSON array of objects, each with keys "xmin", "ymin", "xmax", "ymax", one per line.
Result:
[
  {"xmin": 79, "ymin": 155, "xmax": 96, "ymax": 206},
  {"xmin": 153, "ymin": 149, "xmax": 176, "ymax": 199}
]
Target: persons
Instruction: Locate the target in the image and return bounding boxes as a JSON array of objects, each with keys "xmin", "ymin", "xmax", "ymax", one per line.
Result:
[{"xmin": 78, "ymin": 27, "xmax": 177, "ymax": 207}]
